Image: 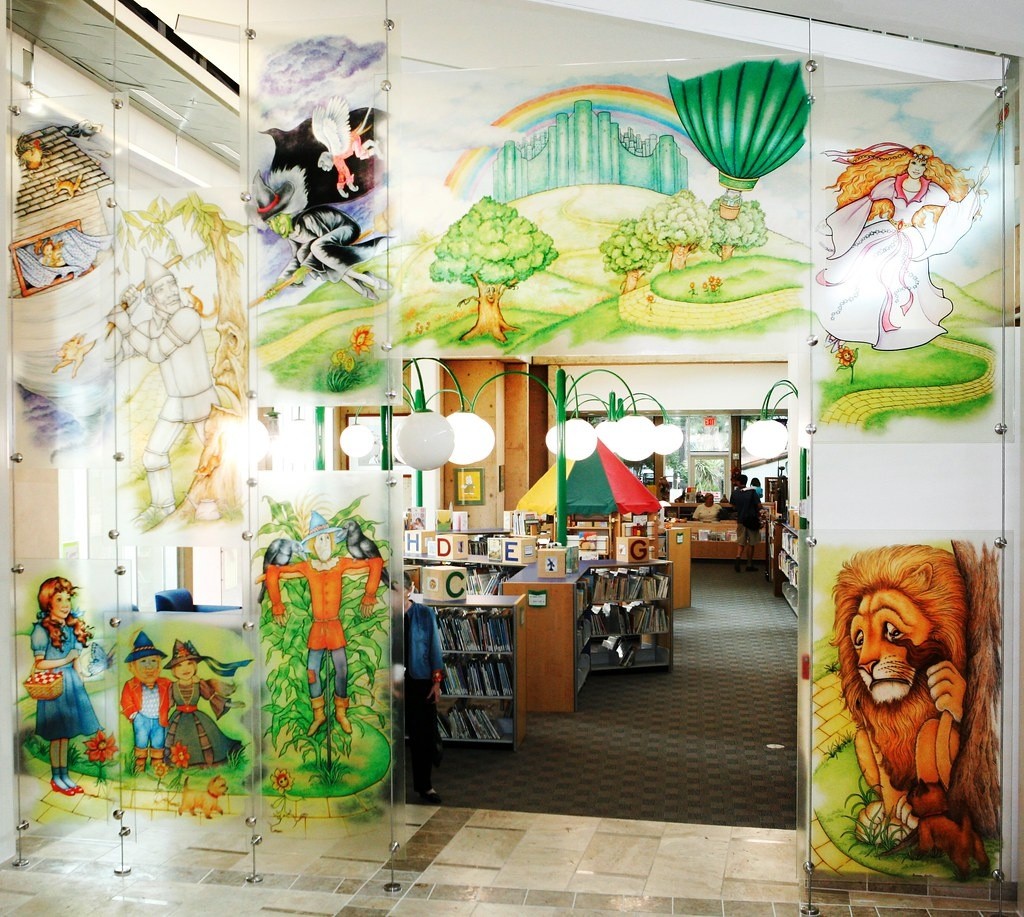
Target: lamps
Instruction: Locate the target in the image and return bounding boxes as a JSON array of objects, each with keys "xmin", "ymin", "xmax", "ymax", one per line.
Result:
[
  {"xmin": 340, "ymin": 359, "xmax": 684, "ymax": 471},
  {"xmin": 743, "ymin": 379, "xmax": 804, "ymax": 461}
]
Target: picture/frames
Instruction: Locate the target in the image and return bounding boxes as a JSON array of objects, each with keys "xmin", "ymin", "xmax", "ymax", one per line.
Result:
[
  {"xmin": 499, "ymin": 465, "xmax": 506, "ymax": 492},
  {"xmin": 454, "ymin": 468, "xmax": 483, "ymax": 505}
]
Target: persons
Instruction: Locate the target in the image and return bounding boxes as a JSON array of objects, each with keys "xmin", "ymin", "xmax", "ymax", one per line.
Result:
[
  {"xmin": 391, "ymin": 573, "xmax": 446, "ymax": 803},
  {"xmin": 692, "ymin": 493, "xmax": 722, "ymax": 521},
  {"xmin": 730, "ymin": 475, "xmax": 762, "ymax": 572},
  {"xmin": 741, "ymin": 477, "xmax": 763, "ymax": 498}
]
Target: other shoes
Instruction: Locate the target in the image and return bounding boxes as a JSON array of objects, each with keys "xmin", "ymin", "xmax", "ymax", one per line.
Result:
[
  {"xmin": 419, "ymin": 791, "xmax": 439, "ymax": 802},
  {"xmin": 735, "ymin": 562, "xmax": 739, "ymax": 571},
  {"xmin": 746, "ymin": 567, "xmax": 759, "ymax": 570}
]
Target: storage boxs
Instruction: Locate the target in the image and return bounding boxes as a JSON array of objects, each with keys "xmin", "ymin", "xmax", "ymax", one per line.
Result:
[
  {"xmin": 788, "ymin": 509, "xmax": 798, "ymax": 527},
  {"xmin": 617, "ymin": 536, "xmax": 649, "ymax": 565},
  {"xmin": 553, "ymin": 546, "xmax": 579, "ymax": 574},
  {"xmin": 437, "ymin": 534, "xmax": 468, "ymax": 560},
  {"xmin": 405, "ymin": 530, "xmax": 436, "ymax": 553},
  {"xmin": 502, "ymin": 538, "xmax": 536, "ymax": 564},
  {"xmin": 793, "ymin": 514, "xmax": 799, "ymax": 529},
  {"xmin": 538, "ymin": 548, "xmax": 566, "ymax": 579},
  {"xmin": 488, "ymin": 538, "xmax": 502, "ymax": 562},
  {"xmin": 422, "ymin": 565, "xmax": 467, "ymax": 604}
]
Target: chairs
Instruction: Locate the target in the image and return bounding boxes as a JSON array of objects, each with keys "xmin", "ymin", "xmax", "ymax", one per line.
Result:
[{"xmin": 154, "ymin": 588, "xmax": 241, "ymax": 613}]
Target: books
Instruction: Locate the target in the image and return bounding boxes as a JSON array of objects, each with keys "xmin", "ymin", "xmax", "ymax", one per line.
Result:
[
  {"xmin": 468, "ymin": 536, "xmax": 501, "ymax": 563},
  {"xmin": 769, "ymin": 507, "xmax": 799, "ymax": 587},
  {"xmin": 433, "ymin": 608, "xmax": 512, "ymax": 739},
  {"xmin": 576, "ymin": 566, "xmax": 670, "ymax": 687},
  {"xmin": 466, "ymin": 566, "xmax": 513, "ymax": 596}
]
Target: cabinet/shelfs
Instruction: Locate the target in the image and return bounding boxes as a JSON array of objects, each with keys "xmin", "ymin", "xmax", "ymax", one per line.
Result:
[{"xmin": 405, "ymin": 502, "xmax": 799, "ymax": 749}]
